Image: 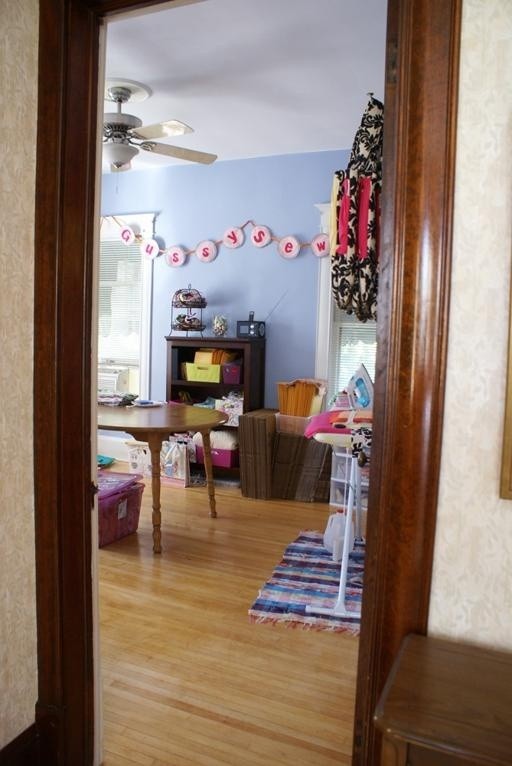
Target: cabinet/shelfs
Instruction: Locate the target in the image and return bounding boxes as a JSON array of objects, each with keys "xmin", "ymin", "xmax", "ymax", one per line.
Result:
[
  {"xmin": 165, "ymin": 335, "xmax": 265, "ymax": 482},
  {"xmin": 328, "ymin": 444, "xmax": 370, "ymax": 511}
]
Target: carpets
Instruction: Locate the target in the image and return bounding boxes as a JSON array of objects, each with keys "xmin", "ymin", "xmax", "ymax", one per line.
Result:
[{"xmin": 249, "ymin": 532, "xmax": 366, "ymax": 639}]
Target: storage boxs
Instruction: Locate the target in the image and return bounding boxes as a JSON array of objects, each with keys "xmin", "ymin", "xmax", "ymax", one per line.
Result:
[
  {"xmin": 98, "ymin": 469, "xmax": 145, "ymax": 547},
  {"xmin": 221, "ymin": 365, "xmax": 241, "ymax": 384},
  {"xmin": 195, "ymin": 445, "xmax": 238, "ymax": 468},
  {"xmin": 215, "ymin": 399, "xmax": 244, "ymax": 427},
  {"xmin": 186, "ymin": 364, "xmax": 221, "ymax": 383}
]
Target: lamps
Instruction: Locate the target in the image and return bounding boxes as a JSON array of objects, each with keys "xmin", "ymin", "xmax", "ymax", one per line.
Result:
[{"xmin": 101, "ymin": 113, "xmax": 155, "ymax": 168}]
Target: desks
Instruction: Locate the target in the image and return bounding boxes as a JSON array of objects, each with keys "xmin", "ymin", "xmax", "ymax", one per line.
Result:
[
  {"xmin": 98, "ymin": 401, "xmax": 229, "ymax": 554},
  {"xmin": 372, "ymin": 631, "xmax": 512, "ymax": 766},
  {"xmin": 306, "ymin": 411, "xmax": 372, "ymax": 619}
]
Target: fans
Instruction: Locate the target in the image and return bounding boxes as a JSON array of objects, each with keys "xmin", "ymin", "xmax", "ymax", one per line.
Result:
[{"xmin": 102, "ymin": 77, "xmax": 218, "ymax": 172}]
